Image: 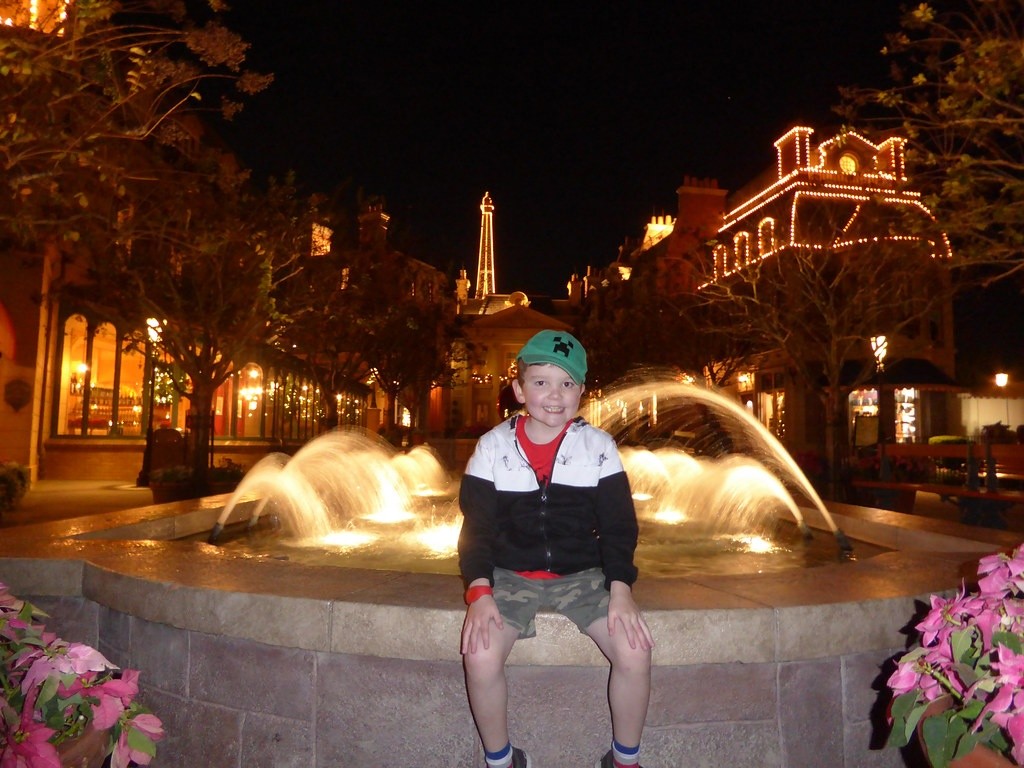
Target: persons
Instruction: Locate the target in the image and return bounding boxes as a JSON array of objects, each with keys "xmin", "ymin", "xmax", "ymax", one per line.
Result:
[{"xmin": 458, "ymin": 329, "xmax": 655, "ymax": 768}]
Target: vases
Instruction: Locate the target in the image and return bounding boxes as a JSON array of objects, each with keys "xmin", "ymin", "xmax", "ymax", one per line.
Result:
[
  {"xmin": 55, "ymin": 706, "xmax": 113, "ymax": 768},
  {"xmin": 917, "ymin": 690, "xmax": 1019, "ymax": 768}
]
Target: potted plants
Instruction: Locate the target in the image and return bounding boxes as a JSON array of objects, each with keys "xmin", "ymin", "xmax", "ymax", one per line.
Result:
[
  {"xmin": 141, "ymin": 465, "xmax": 195, "ymax": 505},
  {"xmin": 207, "ymin": 460, "xmax": 244, "ymax": 495}
]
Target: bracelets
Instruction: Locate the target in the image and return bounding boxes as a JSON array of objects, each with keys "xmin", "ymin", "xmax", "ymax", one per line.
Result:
[{"xmin": 464, "ymin": 586, "xmax": 492, "ymax": 605}]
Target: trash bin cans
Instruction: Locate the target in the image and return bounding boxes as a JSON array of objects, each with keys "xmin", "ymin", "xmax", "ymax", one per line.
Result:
[{"xmin": 151, "ymin": 427, "xmax": 184, "ymax": 472}]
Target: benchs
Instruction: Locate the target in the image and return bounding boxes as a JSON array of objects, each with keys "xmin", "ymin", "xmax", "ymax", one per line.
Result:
[{"xmin": 849, "ymin": 441, "xmax": 1024, "ymax": 532}]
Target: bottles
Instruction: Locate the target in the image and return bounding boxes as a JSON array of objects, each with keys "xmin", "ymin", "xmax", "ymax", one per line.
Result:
[
  {"xmin": 967, "ymin": 439, "xmax": 978, "ymax": 489},
  {"xmin": 984, "ymin": 442, "xmax": 998, "ymax": 492},
  {"xmin": 70, "ymin": 381, "xmax": 144, "ymax": 427}
]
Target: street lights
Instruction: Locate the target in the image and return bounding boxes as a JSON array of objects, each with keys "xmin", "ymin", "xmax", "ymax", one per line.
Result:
[{"xmin": 135, "ymin": 316, "xmax": 169, "ymax": 488}]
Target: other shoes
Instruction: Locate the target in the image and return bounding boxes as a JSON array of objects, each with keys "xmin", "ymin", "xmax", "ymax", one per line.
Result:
[
  {"xmin": 509, "ymin": 746, "xmax": 528, "ymax": 768},
  {"xmin": 595, "ymin": 749, "xmax": 615, "ymax": 768}
]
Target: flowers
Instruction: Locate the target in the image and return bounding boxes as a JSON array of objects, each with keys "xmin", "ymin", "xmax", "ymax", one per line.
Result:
[
  {"xmin": 883, "ymin": 542, "xmax": 1024, "ymax": 768},
  {"xmin": 0, "ymin": 582, "xmax": 170, "ymax": 768}
]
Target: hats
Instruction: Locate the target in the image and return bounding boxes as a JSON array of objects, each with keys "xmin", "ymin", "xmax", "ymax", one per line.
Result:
[{"xmin": 516, "ymin": 330, "xmax": 588, "ymax": 385}]
